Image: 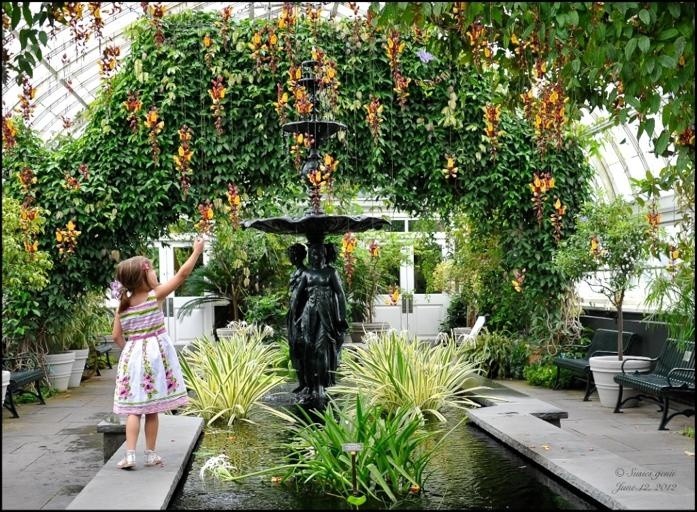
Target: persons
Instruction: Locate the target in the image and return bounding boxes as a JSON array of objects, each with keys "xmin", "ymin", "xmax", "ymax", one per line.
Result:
[
  {"xmin": 285, "ymin": 242, "xmax": 348, "ymax": 405},
  {"xmin": 112, "ymin": 236, "xmax": 205, "ymax": 470}
]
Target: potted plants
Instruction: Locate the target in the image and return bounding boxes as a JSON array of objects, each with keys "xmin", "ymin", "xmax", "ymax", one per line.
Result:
[
  {"xmin": 556, "ymin": 196, "xmax": 676, "ymax": 407},
  {"xmin": 44, "ymin": 259, "xmax": 90, "ymax": 392},
  {"xmin": 178, "ymin": 246, "xmax": 246, "ymax": 343},
  {"xmin": 326, "ymin": 229, "xmax": 426, "ymax": 343}
]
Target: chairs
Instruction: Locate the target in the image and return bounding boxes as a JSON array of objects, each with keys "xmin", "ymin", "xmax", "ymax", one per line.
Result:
[
  {"xmin": 3, "ymin": 367, "xmax": 45, "ymax": 419},
  {"xmin": 93, "ymin": 336, "xmax": 112, "ymax": 375},
  {"xmin": 613, "ymin": 337, "xmax": 695, "ymax": 431},
  {"xmin": 554, "ymin": 329, "xmax": 637, "ymax": 401}
]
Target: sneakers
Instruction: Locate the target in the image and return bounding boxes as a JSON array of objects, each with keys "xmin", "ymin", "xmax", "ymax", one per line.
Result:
[
  {"xmin": 144, "ymin": 450, "xmax": 162, "ymax": 466},
  {"xmin": 117, "ymin": 450, "xmax": 137, "ymax": 469}
]
